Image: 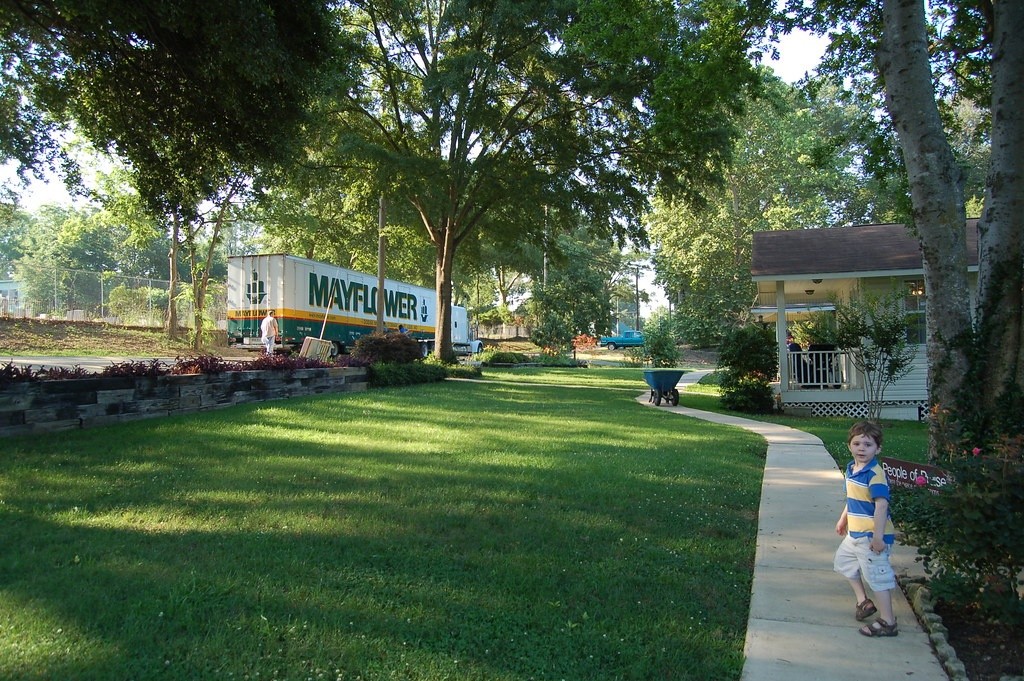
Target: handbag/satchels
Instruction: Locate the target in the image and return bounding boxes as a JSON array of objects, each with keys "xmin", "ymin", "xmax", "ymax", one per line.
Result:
[{"xmin": 260, "ymin": 337, "xmax": 269, "ymax": 345}]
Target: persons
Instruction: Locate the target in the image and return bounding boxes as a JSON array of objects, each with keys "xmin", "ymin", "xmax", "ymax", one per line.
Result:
[
  {"xmin": 261, "ymin": 311, "xmax": 279, "ymax": 355},
  {"xmin": 834, "ymin": 421, "xmax": 898, "ymax": 637},
  {"xmin": 399, "ymin": 324, "xmax": 408, "ymax": 334}
]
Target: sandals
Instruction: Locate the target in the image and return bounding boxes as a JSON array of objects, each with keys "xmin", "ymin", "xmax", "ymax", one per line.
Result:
[
  {"xmin": 859, "ymin": 617, "xmax": 899, "ymax": 637},
  {"xmin": 856, "ymin": 599, "xmax": 877, "ymax": 620}
]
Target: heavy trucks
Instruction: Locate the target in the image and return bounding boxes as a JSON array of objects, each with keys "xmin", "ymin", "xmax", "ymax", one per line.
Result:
[{"xmin": 226, "ymin": 253, "xmax": 485, "ymax": 358}]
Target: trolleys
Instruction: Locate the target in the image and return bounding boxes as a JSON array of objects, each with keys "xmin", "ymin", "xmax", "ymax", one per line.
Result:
[{"xmin": 642, "ymin": 370, "xmax": 684, "ymax": 406}]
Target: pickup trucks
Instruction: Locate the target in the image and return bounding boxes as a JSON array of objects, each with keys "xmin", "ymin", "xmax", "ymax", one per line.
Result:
[{"xmin": 600, "ymin": 330, "xmax": 647, "ymax": 350}]
